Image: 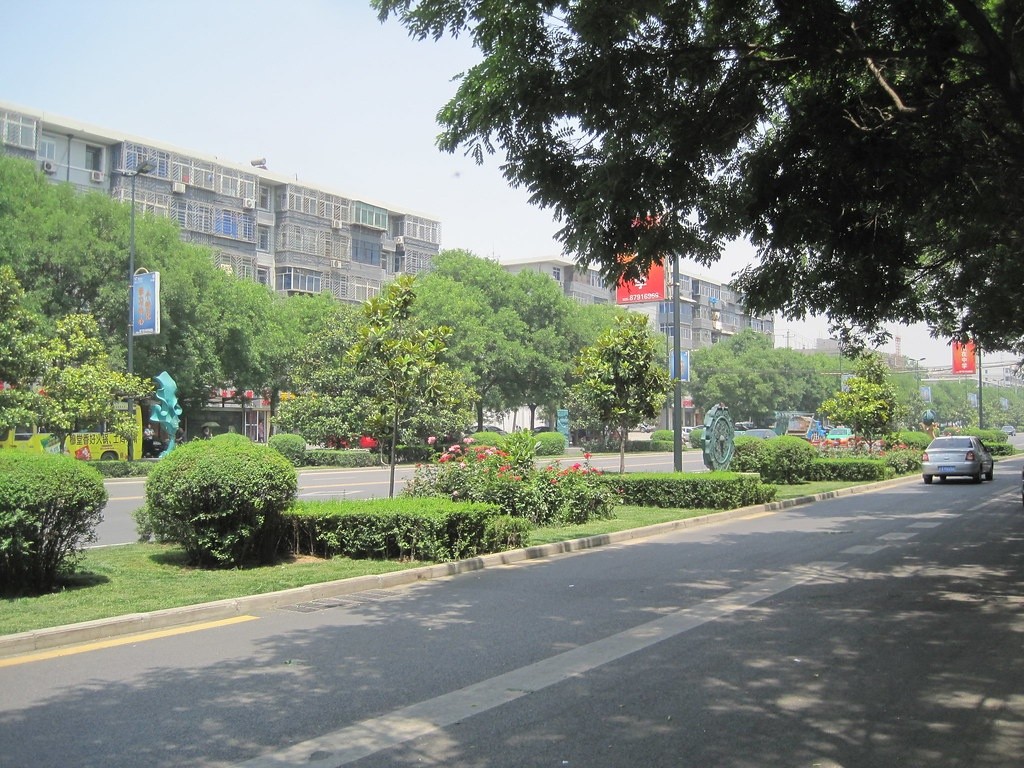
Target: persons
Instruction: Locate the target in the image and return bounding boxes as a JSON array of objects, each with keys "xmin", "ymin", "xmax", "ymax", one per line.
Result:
[
  {"xmin": 258, "ymin": 421, "xmax": 263, "ymax": 442},
  {"xmin": 143, "ymin": 422, "xmax": 155, "ymax": 458},
  {"xmin": 175, "ymin": 426, "xmax": 188, "ymax": 442},
  {"xmin": 203, "ymin": 428, "xmax": 210, "ymax": 441}
]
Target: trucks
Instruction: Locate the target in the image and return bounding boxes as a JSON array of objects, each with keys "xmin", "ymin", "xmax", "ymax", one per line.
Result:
[{"xmin": 784, "ymin": 412, "xmax": 826, "ymax": 442}]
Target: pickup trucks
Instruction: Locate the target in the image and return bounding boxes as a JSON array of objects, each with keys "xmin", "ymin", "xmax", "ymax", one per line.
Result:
[{"xmin": 826, "ymin": 428, "xmax": 854, "ymax": 440}]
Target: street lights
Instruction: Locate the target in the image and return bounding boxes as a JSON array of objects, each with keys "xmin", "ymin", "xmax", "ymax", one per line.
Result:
[
  {"xmin": 998, "ymin": 378, "xmax": 1004, "ymax": 404},
  {"xmin": 909, "ymin": 357, "xmax": 926, "ymax": 390},
  {"xmin": 111, "ymin": 159, "xmax": 159, "ymax": 462}
]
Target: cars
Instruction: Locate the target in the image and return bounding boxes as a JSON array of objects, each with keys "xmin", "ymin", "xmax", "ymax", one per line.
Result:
[
  {"xmin": 534, "ymin": 427, "xmax": 558, "ymax": 433},
  {"xmin": 1001, "ymin": 425, "xmax": 1016, "ymax": 436},
  {"xmin": 745, "ymin": 428, "xmax": 779, "ymax": 438},
  {"xmin": 921, "ymin": 436, "xmax": 994, "ymax": 485},
  {"xmin": 627, "ymin": 421, "xmax": 654, "ymax": 433},
  {"xmin": 470, "ymin": 425, "xmax": 507, "ymax": 435},
  {"xmin": 732, "ymin": 431, "xmax": 745, "ymax": 440},
  {"xmin": 682, "ymin": 424, "xmax": 705, "ymax": 443}
]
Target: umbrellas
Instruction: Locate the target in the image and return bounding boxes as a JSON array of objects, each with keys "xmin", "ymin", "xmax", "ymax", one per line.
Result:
[{"xmin": 199, "ymin": 421, "xmax": 220, "ymax": 427}]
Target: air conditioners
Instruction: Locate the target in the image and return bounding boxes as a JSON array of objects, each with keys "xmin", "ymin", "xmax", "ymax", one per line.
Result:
[
  {"xmin": 90, "ymin": 171, "xmax": 104, "ymax": 183},
  {"xmin": 331, "ymin": 260, "xmax": 341, "ymax": 269},
  {"xmin": 171, "ymin": 182, "xmax": 185, "ymax": 194},
  {"xmin": 393, "ymin": 236, "xmax": 404, "ymax": 244},
  {"xmin": 243, "ymin": 198, "xmax": 256, "ymax": 210},
  {"xmin": 331, "ymin": 219, "xmax": 342, "ymax": 229},
  {"xmin": 43, "ymin": 161, "xmax": 57, "ymax": 174}
]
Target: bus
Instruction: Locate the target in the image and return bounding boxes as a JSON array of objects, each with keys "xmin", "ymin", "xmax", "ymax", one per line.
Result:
[
  {"xmin": 0, "ymin": 401, "xmax": 143, "ymax": 464},
  {"xmin": 735, "ymin": 422, "xmax": 755, "ymax": 430}
]
